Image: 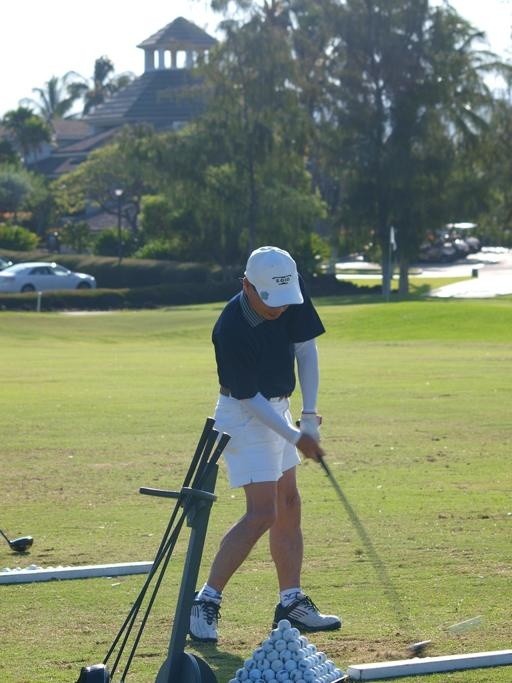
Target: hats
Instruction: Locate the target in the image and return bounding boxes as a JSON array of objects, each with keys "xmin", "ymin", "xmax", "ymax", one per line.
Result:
[{"xmin": 245, "ymin": 245, "xmax": 304, "ymax": 307}]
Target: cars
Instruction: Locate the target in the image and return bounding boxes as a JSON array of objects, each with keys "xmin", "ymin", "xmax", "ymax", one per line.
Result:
[{"xmin": 0, "ymin": 261, "xmax": 97, "ymax": 293}]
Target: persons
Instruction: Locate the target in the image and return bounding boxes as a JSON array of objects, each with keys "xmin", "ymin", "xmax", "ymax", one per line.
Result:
[{"xmin": 186, "ymin": 246, "xmax": 341, "ymax": 644}]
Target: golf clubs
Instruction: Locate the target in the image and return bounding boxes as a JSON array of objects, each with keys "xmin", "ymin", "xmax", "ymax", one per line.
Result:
[
  {"xmin": 1, "ymin": 531, "xmax": 32, "ymax": 552},
  {"xmin": 79, "ymin": 417, "xmax": 232, "ymax": 682},
  {"xmin": 296, "ymin": 418, "xmax": 430, "ymax": 654}
]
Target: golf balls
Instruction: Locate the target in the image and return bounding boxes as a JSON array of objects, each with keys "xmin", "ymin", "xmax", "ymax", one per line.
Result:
[{"xmin": 228, "ymin": 619, "xmax": 346, "ymax": 683}]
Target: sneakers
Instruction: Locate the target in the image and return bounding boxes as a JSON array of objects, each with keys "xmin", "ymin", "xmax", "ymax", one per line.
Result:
[
  {"xmin": 189, "ymin": 590, "xmax": 222, "ymax": 642},
  {"xmin": 272, "ymin": 592, "xmax": 341, "ymax": 633}
]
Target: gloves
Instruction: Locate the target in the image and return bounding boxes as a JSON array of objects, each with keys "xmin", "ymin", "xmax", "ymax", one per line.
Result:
[{"xmin": 300, "ymin": 412, "xmax": 321, "ymax": 443}]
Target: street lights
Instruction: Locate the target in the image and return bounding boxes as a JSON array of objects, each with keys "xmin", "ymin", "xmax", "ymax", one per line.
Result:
[{"xmin": 114, "ymin": 187, "xmax": 126, "ymax": 257}]
